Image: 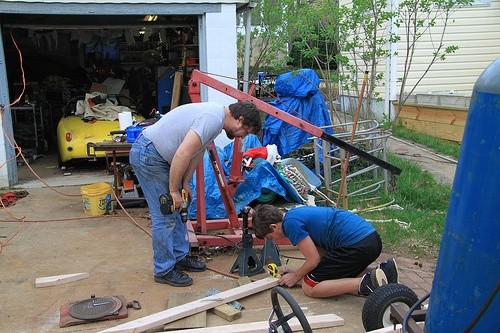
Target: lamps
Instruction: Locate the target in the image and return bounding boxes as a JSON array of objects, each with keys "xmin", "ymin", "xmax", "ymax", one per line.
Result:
[{"xmin": 143, "ymin": 15, "xmax": 158, "ymax": 22}]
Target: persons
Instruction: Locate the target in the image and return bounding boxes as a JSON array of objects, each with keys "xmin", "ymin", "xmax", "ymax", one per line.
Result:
[
  {"xmin": 250, "ymin": 198, "xmax": 400, "ymax": 301},
  {"xmin": 127, "ymin": 98, "xmax": 264, "ymax": 288}
]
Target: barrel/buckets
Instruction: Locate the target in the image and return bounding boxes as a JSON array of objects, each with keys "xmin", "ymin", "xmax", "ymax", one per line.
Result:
[{"xmin": 81, "ymin": 183, "xmax": 114, "ymax": 216}]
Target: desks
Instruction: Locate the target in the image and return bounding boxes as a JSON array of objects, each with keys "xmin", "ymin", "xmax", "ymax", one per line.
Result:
[{"xmin": 10, "ymin": 105, "xmax": 42, "ymax": 149}]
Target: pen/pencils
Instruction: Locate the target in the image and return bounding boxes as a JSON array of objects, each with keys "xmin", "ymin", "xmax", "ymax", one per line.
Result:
[{"xmin": 282, "ymin": 258, "xmax": 289, "ymax": 276}]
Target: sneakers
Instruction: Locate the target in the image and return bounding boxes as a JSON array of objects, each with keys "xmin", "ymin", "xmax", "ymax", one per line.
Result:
[
  {"xmin": 362, "ymin": 269, "xmax": 388, "ymax": 295},
  {"xmin": 379, "ymin": 257, "xmax": 399, "ymax": 283}
]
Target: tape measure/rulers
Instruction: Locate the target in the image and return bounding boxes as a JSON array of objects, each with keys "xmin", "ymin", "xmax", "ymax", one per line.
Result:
[{"xmin": 267, "ymin": 263, "xmax": 281, "ymax": 278}]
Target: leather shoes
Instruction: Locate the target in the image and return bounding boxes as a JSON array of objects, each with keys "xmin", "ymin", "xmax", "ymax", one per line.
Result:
[
  {"xmin": 154, "ymin": 267, "xmax": 193, "ymax": 287},
  {"xmin": 175, "ymin": 254, "xmax": 206, "ymax": 271}
]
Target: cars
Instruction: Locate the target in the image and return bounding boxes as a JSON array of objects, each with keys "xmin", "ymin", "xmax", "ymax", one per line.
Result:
[{"xmin": 56, "ymin": 94, "xmax": 147, "ymax": 170}]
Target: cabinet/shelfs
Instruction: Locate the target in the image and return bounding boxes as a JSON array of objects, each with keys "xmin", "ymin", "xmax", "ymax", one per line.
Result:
[
  {"xmin": 171, "ymin": 44, "xmax": 200, "ymax": 103},
  {"xmin": 118, "ymin": 41, "xmax": 156, "ymax": 65}
]
